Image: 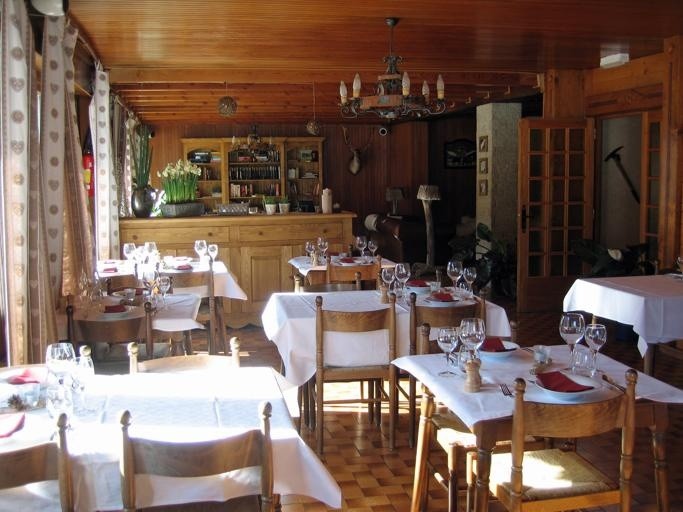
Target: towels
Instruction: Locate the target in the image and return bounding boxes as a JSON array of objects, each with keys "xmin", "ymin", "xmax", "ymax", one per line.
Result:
[
  {"xmin": 105, "ymin": 305, "xmax": 126, "ymax": 314},
  {"xmin": 432, "ymin": 292, "xmax": 452, "ymax": 301},
  {"xmin": 1, "ymin": 414, "xmax": 26, "ymax": 438},
  {"xmin": 535, "ymin": 372, "xmax": 593, "ymax": 393},
  {"xmin": 407, "ymin": 281, "xmax": 427, "ymax": 286},
  {"xmin": 4, "ymin": 370, "xmax": 41, "ymax": 385},
  {"xmin": 340, "ymin": 258, "xmax": 354, "ymax": 264},
  {"xmin": 103, "ymin": 268, "xmax": 117, "ymax": 272},
  {"xmin": 178, "ymin": 265, "xmax": 192, "ymax": 270},
  {"xmin": 480, "ymin": 337, "xmax": 516, "ymax": 352}
]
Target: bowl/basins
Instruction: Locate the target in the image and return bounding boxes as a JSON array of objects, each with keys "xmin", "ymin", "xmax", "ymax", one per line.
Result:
[
  {"xmin": 97, "ymin": 307, "xmax": 132, "ymax": 318},
  {"xmin": 331, "ymin": 256, "xmax": 340, "ymax": 262},
  {"xmin": 353, "ymin": 260, "xmax": 366, "ymax": 266},
  {"xmin": 165, "ymin": 257, "xmax": 193, "ymax": 266},
  {"xmin": 341, "ymin": 253, "xmax": 348, "ymax": 257}
]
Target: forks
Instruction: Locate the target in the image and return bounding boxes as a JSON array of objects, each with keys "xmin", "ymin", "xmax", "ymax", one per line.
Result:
[
  {"xmin": 424, "ymin": 300, "xmax": 431, "ymax": 304},
  {"xmin": 497, "ymin": 383, "xmax": 515, "ymax": 400},
  {"xmin": 523, "ymin": 347, "xmax": 534, "ymax": 353},
  {"xmin": 444, "ymin": 355, "xmax": 466, "ymax": 374}
]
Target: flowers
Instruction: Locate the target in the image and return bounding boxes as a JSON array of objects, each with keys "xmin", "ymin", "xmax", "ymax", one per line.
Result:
[{"xmin": 157, "ymin": 158, "xmax": 201, "ymax": 204}]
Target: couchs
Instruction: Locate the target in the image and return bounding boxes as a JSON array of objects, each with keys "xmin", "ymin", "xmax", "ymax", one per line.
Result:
[{"xmin": 365, "ymin": 213, "xmax": 455, "ymax": 264}]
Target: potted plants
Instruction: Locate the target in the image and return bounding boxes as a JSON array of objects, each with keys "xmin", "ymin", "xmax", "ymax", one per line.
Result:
[
  {"xmin": 265, "ymin": 196, "xmax": 276, "ymax": 216},
  {"xmin": 279, "ymin": 196, "xmax": 290, "ymax": 215},
  {"xmin": 130, "ymin": 124, "xmax": 153, "ymax": 218}
]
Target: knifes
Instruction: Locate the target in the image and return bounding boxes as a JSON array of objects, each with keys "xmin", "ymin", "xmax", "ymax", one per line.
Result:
[
  {"xmin": 451, "ymin": 352, "xmax": 467, "ymax": 363},
  {"xmin": 602, "ymin": 375, "xmax": 626, "ymax": 392}
]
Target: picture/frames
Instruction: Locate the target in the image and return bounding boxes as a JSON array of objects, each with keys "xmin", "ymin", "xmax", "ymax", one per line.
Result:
[
  {"xmin": 479, "ymin": 158, "xmax": 488, "ymax": 174},
  {"xmin": 445, "ymin": 141, "xmax": 476, "ymax": 168},
  {"xmin": 478, "ymin": 180, "xmax": 488, "ymax": 195},
  {"xmin": 479, "ymin": 136, "xmax": 488, "ymax": 152}
]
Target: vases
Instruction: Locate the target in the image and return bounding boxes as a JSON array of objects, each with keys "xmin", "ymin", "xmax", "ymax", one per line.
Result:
[{"xmin": 161, "ymin": 205, "xmax": 202, "ymax": 217}]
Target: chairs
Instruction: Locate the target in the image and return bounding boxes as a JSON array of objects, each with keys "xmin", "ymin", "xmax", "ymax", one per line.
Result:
[
  {"xmin": 420, "ymin": 320, "xmax": 551, "ymax": 512},
  {"xmin": 0, "ymin": 413, "xmax": 74, "ymax": 512},
  {"xmin": 466, "ymin": 369, "xmax": 638, "ymax": 512},
  {"xmin": 121, "ymin": 402, "xmax": 275, "ymax": 512},
  {"xmin": 294, "ymin": 272, "xmax": 374, "ymax": 426},
  {"xmin": 66, "ymin": 302, "xmax": 153, "ymax": 359},
  {"xmin": 107, "ymin": 278, "xmax": 174, "ymax": 295},
  {"xmin": 0, "ymin": 345, "xmax": 90, "ymax": 372},
  {"xmin": 96, "ymin": 262, "xmax": 137, "ymax": 292},
  {"xmin": 154, "ymin": 259, "xmax": 216, "ymax": 354},
  {"xmin": 128, "ymin": 337, "xmax": 241, "ymax": 373},
  {"xmin": 395, "ymin": 290, "xmax": 486, "ymax": 449},
  {"xmin": 377, "ymin": 268, "xmax": 442, "ymax": 289},
  {"xmin": 308, "ymin": 293, "xmax": 395, "ymax": 456},
  {"xmin": 326, "ymin": 255, "xmax": 381, "ymax": 283}
]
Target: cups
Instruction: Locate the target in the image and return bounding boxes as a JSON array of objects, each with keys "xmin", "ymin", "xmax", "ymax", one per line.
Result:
[
  {"xmin": 382, "ymin": 268, "xmax": 395, "ymax": 296},
  {"xmin": 249, "ymin": 207, "xmax": 258, "ymax": 215},
  {"xmin": 19, "ymin": 384, "xmax": 40, "ymax": 409},
  {"xmin": 305, "ymin": 240, "xmax": 317, "ymax": 264},
  {"xmin": 90, "ymin": 289, "xmax": 103, "ymax": 316},
  {"xmin": 319, "ymin": 253, "xmax": 327, "ymax": 266},
  {"xmin": 69, "ymin": 357, "xmax": 94, "ymax": 416},
  {"xmin": 463, "ymin": 267, "xmax": 477, "ymax": 291},
  {"xmin": 124, "ymin": 288, "xmax": 136, "ymax": 302},
  {"xmin": 355, "ymin": 236, "xmax": 367, "ymax": 262},
  {"xmin": 458, "ymin": 345, "xmax": 479, "ymax": 374},
  {"xmin": 368, "ymin": 241, "xmax": 378, "ymax": 263},
  {"xmin": 46, "ymin": 342, "xmax": 76, "ymax": 391},
  {"xmin": 124, "ymin": 243, "xmax": 137, "ymax": 261},
  {"xmin": 46, "ymin": 386, "xmax": 74, "ymax": 434},
  {"xmin": 317, "ymin": 237, "xmax": 328, "ymax": 263},
  {"xmin": 430, "ymin": 281, "xmax": 441, "ymax": 297},
  {"xmin": 144, "ymin": 242, "xmax": 157, "ymax": 263},
  {"xmin": 585, "ymin": 324, "xmax": 607, "ymax": 374},
  {"xmin": 156, "ymin": 276, "xmax": 170, "ymax": 310},
  {"xmin": 459, "ymin": 282, "xmax": 473, "ymax": 300},
  {"xmin": 143, "ymin": 271, "xmax": 155, "ymax": 303},
  {"xmin": 534, "ymin": 346, "xmax": 550, "ymax": 364},
  {"xmin": 460, "ymin": 318, "xmax": 485, "ymax": 360},
  {"xmin": 138, "ymin": 246, "xmax": 148, "ymax": 264},
  {"xmin": 208, "ymin": 244, "xmax": 218, "ymax": 265},
  {"xmin": 394, "ymin": 281, "xmax": 402, "ymax": 297},
  {"xmin": 314, "ymin": 205, "xmax": 320, "ymax": 215},
  {"xmin": 131, "ymin": 250, "xmax": 142, "ymax": 264},
  {"xmin": 363, "ymin": 250, "xmax": 371, "ymax": 263},
  {"xmin": 395, "ymin": 263, "xmax": 411, "ymax": 296},
  {"xmin": 572, "ymin": 348, "xmax": 596, "ymax": 377},
  {"xmin": 194, "ymin": 240, "xmax": 208, "ymax": 265},
  {"xmin": 447, "ymin": 261, "xmax": 463, "ymax": 296},
  {"xmin": 559, "ymin": 313, "xmax": 585, "ymax": 372},
  {"xmin": 437, "ymin": 328, "xmax": 459, "ymax": 378}
]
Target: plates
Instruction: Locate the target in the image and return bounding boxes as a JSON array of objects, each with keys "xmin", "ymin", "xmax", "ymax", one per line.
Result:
[
  {"xmin": 123, "ymin": 305, "xmax": 137, "ymax": 311},
  {"xmin": 426, "ymin": 298, "xmax": 459, "ymax": 302},
  {"xmin": 340, "ymin": 262, "xmax": 361, "ymax": 266},
  {"xmin": 112, "ymin": 291, "xmax": 123, "ymax": 297},
  {"xmin": 0, "ymin": 368, "xmax": 46, "ymax": 384},
  {"xmin": 476, "ymin": 341, "xmax": 520, "ymax": 359},
  {"xmin": 535, "ymin": 375, "xmax": 602, "ymax": 399}
]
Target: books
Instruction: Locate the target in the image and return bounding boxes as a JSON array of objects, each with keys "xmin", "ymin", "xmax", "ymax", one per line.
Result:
[
  {"xmin": 209, "ymin": 151, "xmax": 220, "ymax": 163},
  {"xmin": 268, "ymin": 182, "xmax": 280, "ymax": 196},
  {"xmin": 230, "ymin": 183, "xmax": 256, "ymax": 197}
]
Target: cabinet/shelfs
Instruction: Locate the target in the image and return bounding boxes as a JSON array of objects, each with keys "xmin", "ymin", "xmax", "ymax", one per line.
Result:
[
  {"xmin": 225, "ymin": 138, "xmax": 284, "ymax": 212},
  {"xmin": 284, "ymin": 138, "xmax": 325, "ymax": 212},
  {"xmin": 119, "ymin": 217, "xmax": 235, "ymax": 329},
  {"xmin": 236, "ymin": 212, "xmax": 357, "ymax": 329},
  {"xmin": 181, "ymin": 137, "xmax": 225, "ymax": 212}
]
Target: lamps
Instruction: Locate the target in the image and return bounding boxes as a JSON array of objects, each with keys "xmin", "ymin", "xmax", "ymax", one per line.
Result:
[
  {"xmin": 306, "ymin": 81, "xmax": 324, "ymax": 136},
  {"xmin": 231, "ymin": 125, "xmax": 276, "ymax": 163},
  {"xmin": 339, "ymin": 17, "xmax": 445, "ymax": 121},
  {"xmin": 217, "ymin": 82, "xmax": 237, "ymax": 117}
]
